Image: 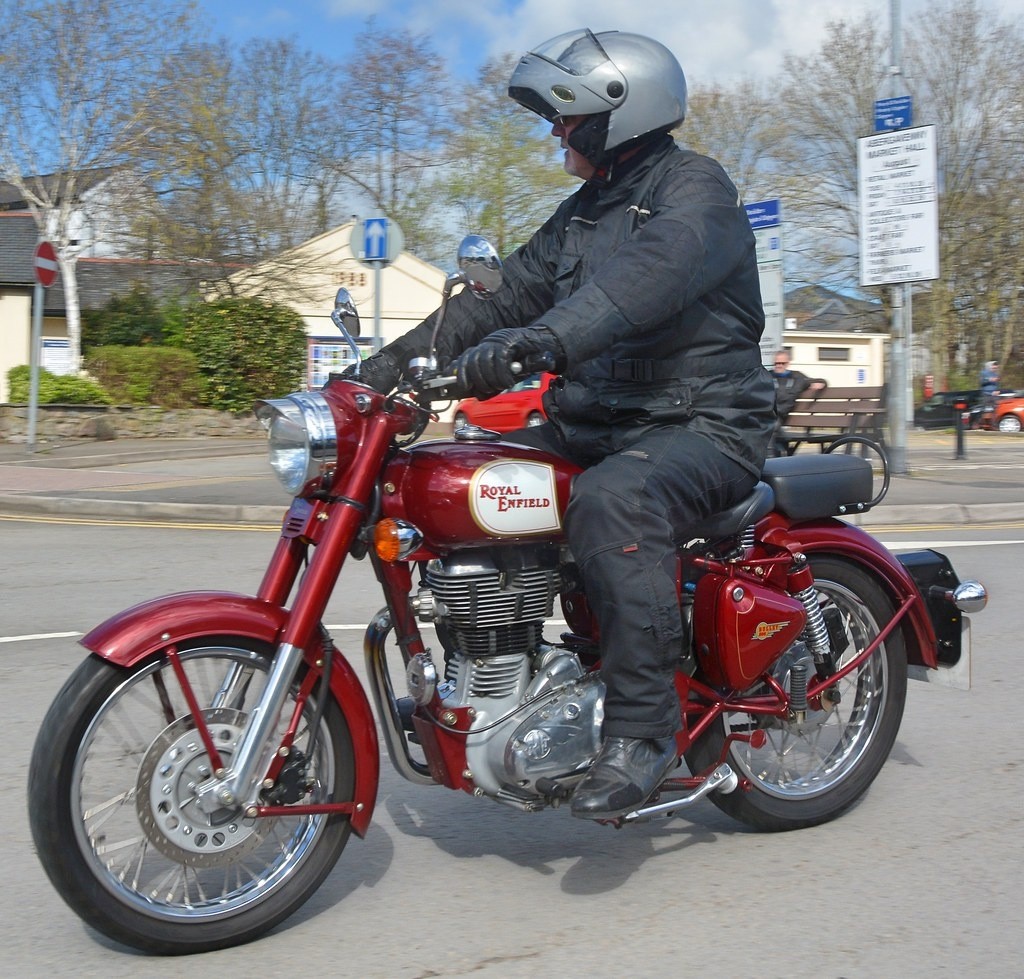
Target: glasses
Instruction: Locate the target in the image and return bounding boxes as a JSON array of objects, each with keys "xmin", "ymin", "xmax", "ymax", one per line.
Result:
[{"xmin": 775, "ymin": 360, "xmax": 789, "ymax": 365}]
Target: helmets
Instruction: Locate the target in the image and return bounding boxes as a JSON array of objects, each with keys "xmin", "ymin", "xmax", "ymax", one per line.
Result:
[{"xmin": 508, "ymin": 27, "xmax": 688, "ymax": 165}]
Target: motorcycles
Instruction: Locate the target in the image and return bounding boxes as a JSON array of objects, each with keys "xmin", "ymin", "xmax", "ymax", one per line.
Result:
[{"xmin": 28, "ymin": 230, "xmax": 990, "ymax": 958}]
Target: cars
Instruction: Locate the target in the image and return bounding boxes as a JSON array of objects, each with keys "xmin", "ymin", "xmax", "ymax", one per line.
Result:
[
  {"xmin": 982, "ymin": 390, "xmax": 1024, "ymax": 434},
  {"xmin": 961, "ymin": 393, "xmax": 1013, "ymax": 429}
]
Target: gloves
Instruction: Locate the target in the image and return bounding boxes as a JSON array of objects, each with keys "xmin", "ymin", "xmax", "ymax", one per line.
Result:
[
  {"xmin": 455, "ymin": 327, "xmax": 564, "ymax": 397},
  {"xmin": 336, "ymin": 354, "xmax": 399, "ymax": 391}
]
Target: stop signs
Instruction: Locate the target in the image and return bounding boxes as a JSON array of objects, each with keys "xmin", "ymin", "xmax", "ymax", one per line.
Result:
[{"xmin": 32, "ymin": 242, "xmax": 60, "ymax": 289}]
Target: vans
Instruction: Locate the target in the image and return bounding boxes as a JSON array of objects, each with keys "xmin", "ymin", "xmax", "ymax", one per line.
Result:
[{"xmin": 914, "ymin": 389, "xmax": 988, "ymax": 430}]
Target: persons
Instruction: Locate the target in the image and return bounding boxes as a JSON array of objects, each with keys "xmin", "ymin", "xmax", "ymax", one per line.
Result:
[
  {"xmin": 765, "ymin": 348, "xmax": 809, "ymax": 458},
  {"xmin": 968, "ymin": 360, "xmax": 1005, "ymax": 432},
  {"xmin": 314, "ymin": 26, "xmax": 782, "ymax": 824}
]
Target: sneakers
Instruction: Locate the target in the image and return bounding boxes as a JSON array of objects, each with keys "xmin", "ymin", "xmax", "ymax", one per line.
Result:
[{"xmin": 566, "ymin": 732, "xmax": 679, "ymax": 819}]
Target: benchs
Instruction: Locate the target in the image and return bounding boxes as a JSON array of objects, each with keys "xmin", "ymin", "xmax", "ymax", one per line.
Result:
[{"xmin": 777, "ymin": 382, "xmax": 891, "ymax": 459}]
